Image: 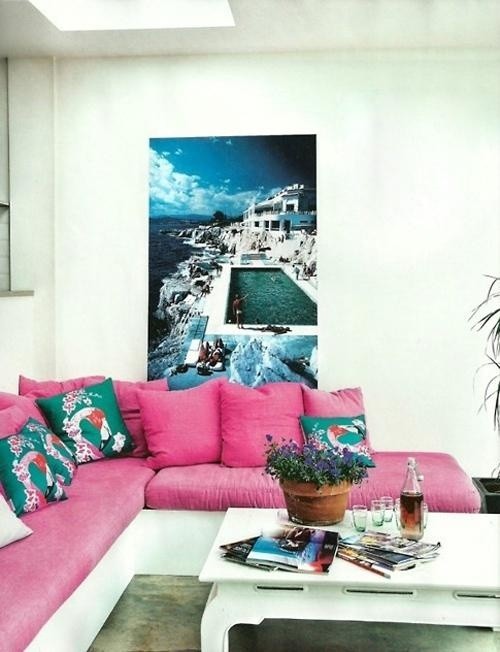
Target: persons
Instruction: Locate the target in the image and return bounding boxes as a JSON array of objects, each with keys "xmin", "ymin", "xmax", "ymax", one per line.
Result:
[{"xmin": 187, "ymin": 234, "xmax": 317, "ymax": 367}]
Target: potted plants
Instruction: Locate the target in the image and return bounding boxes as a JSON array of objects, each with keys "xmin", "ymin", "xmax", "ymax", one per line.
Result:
[{"xmin": 467, "ymin": 271, "xmax": 500, "ymax": 515}]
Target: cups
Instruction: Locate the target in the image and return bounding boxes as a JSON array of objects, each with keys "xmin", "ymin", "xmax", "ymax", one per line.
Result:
[
  {"xmin": 370, "ymin": 495, "xmax": 428, "ymax": 526},
  {"xmin": 353, "ymin": 504, "xmax": 368, "ymax": 534}
]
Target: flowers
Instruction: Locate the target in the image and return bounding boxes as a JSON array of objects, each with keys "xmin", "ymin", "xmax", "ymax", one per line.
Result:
[{"xmin": 253, "ymin": 426, "xmax": 378, "ymax": 493}]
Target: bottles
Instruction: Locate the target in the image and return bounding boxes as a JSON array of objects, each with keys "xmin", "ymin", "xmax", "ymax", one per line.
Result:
[{"xmin": 399, "ymin": 457, "xmax": 423, "ymax": 541}]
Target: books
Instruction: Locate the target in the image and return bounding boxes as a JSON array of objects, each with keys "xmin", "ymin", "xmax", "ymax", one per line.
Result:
[{"xmin": 218, "ymin": 520, "xmax": 443, "ymax": 580}]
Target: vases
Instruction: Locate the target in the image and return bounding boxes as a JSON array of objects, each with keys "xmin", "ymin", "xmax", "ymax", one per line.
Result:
[{"xmin": 274, "ymin": 468, "xmax": 354, "ymax": 527}]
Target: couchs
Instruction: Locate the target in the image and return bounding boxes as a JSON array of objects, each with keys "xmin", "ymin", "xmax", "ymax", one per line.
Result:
[{"xmin": 0, "ymin": 373, "xmax": 482, "ymax": 652}]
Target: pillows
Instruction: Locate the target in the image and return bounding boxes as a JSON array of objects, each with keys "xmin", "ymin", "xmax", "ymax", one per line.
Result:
[
  {"xmin": 217, "ymin": 380, "xmax": 306, "ymax": 469},
  {"xmin": 131, "ymin": 375, "xmax": 232, "ymax": 471},
  {"xmin": 0, "ymin": 430, "xmax": 69, "ymax": 517},
  {"xmin": 17, "ymin": 416, "xmax": 79, "ymax": 488},
  {"xmin": 297, "ymin": 414, "xmax": 376, "ymax": 470},
  {"xmin": 0, "ymin": 493, "xmax": 33, "ymax": 552},
  {"xmin": 0, "ymin": 375, "xmax": 169, "ymax": 466},
  {"xmin": 300, "ymin": 383, "xmax": 376, "ymax": 455}
]
